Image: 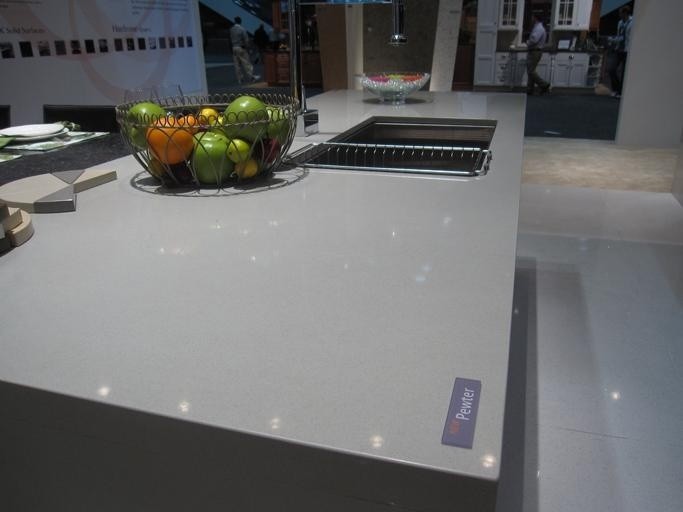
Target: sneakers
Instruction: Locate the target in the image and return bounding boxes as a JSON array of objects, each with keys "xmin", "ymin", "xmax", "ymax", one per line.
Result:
[
  {"xmin": 238, "ymin": 74, "xmax": 261, "ymax": 85},
  {"xmin": 525, "ymin": 84, "xmax": 551, "ymax": 95}
]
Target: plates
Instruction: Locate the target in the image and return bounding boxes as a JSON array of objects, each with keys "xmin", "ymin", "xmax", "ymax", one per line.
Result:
[{"xmin": 0, "ymin": 124, "xmax": 70, "ymax": 142}]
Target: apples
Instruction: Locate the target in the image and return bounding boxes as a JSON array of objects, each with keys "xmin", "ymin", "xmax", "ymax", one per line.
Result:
[
  {"xmin": 126, "ymin": 103, "xmax": 166, "ymax": 150},
  {"xmin": 193, "ymin": 95, "xmax": 292, "ymax": 183}
]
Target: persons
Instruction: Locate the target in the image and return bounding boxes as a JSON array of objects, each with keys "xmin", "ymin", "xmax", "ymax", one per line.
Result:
[
  {"xmin": 607, "ymin": 4, "xmax": 631, "ymax": 98},
  {"xmin": 516, "ymin": 9, "xmax": 552, "ymax": 93},
  {"xmin": 227, "ymin": 13, "xmax": 317, "ymax": 87}
]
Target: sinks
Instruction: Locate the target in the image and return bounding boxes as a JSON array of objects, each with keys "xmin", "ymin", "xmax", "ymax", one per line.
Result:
[{"xmin": 289, "ymin": 115, "xmax": 498, "ymax": 177}]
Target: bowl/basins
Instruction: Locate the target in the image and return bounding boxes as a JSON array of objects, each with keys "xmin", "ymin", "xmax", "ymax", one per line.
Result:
[{"xmin": 356, "ymin": 71, "xmax": 430, "ymax": 104}]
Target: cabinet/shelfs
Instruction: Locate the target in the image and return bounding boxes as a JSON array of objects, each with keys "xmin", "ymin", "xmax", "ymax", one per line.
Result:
[{"xmin": 472, "ymin": 0, "xmax": 605, "ymax": 88}]
